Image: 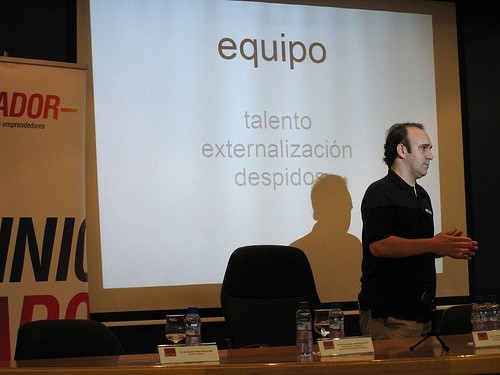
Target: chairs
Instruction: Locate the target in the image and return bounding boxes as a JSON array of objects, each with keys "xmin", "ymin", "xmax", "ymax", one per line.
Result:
[
  {"xmin": 221, "ymin": 245, "xmax": 323, "ymax": 347},
  {"xmin": 14, "ymin": 320, "xmax": 126, "ymax": 361}
]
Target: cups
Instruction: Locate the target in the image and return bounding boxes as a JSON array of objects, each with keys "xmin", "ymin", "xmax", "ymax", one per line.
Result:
[{"xmin": 165, "ymin": 315, "xmax": 186, "ymax": 344}]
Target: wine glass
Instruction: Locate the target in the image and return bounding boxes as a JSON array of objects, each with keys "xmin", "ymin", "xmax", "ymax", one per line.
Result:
[{"xmin": 314, "ymin": 309, "xmax": 330, "ymax": 356}]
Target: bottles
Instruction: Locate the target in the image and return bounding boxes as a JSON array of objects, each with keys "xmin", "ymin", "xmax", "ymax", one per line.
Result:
[
  {"xmin": 471, "ymin": 296, "xmax": 489, "ymax": 331},
  {"xmin": 485, "ymin": 294, "xmax": 500, "ymax": 331},
  {"xmin": 328, "ymin": 302, "xmax": 345, "ymax": 339},
  {"xmin": 184, "ymin": 306, "xmax": 202, "ymax": 344},
  {"xmin": 296, "ymin": 301, "xmax": 313, "ymax": 357}
]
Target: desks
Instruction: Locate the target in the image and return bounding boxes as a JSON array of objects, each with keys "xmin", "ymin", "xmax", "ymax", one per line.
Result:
[{"xmin": 0, "ymin": 334, "xmax": 500, "ymax": 375}]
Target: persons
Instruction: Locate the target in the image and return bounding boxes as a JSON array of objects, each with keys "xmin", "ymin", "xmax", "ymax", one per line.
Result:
[{"xmin": 358, "ymin": 122, "xmax": 479, "ymax": 339}]
